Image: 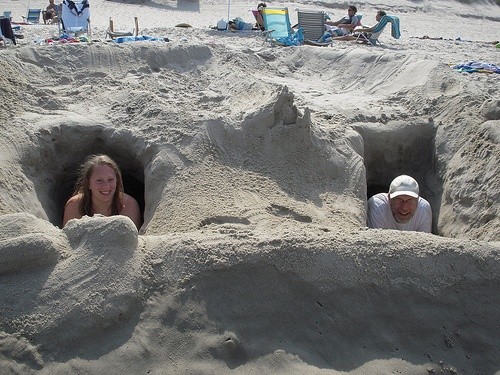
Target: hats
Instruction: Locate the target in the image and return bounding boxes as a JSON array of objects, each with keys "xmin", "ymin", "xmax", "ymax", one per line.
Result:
[{"xmin": 389, "ymin": 175, "xmax": 420, "ymax": 199}]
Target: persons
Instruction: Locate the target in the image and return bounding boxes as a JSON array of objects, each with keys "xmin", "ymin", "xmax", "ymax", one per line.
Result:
[
  {"xmin": 368, "ymin": 174, "xmax": 433, "ymax": 233},
  {"xmin": 63, "ymin": 154, "xmax": 140, "ymax": 229},
  {"xmin": 324, "ymin": 6, "xmax": 387, "ymax": 44},
  {"xmin": 45, "ymin": 0, "xmax": 62, "ymax": 23},
  {"xmin": 255, "ymin": 2, "xmax": 267, "ymax": 32},
  {"xmin": 228, "ymin": 20, "xmax": 261, "ymax": 32}
]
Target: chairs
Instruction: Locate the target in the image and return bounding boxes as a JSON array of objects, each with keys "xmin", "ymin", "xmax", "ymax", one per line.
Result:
[
  {"xmin": 251, "ymin": 10, "xmax": 401, "ymax": 48},
  {"xmin": 0, "ymin": 0, "xmax": 91, "ymax": 49},
  {"xmin": 105, "ymin": 16, "xmax": 138, "ymax": 40}
]
